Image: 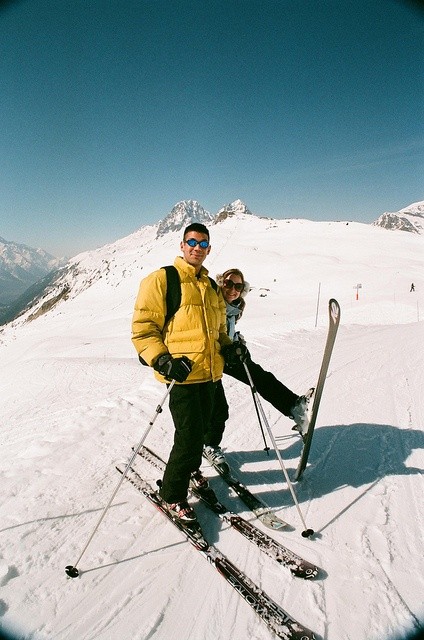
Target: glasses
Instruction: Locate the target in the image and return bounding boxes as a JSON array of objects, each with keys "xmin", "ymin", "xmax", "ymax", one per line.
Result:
[
  {"xmin": 223, "ymin": 279, "xmax": 243, "ymax": 292},
  {"xmin": 185, "ymin": 239, "xmax": 209, "ymax": 248}
]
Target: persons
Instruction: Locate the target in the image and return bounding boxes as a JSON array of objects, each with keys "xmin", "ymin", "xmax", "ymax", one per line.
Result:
[
  {"xmin": 202, "ymin": 269, "xmax": 314, "ymax": 463},
  {"xmin": 130, "ymin": 222, "xmax": 252, "ymax": 525}
]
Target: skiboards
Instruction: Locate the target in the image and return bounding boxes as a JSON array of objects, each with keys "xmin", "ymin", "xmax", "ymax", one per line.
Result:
[
  {"xmin": 115, "ymin": 441, "xmax": 320, "ymax": 640},
  {"xmin": 202, "ymin": 298, "xmax": 340, "ymax": 532}
]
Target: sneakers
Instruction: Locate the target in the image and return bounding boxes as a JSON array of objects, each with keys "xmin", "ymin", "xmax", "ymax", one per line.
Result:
[
  {"xmin": 156, "ymin": 492, "xmax": 197, "ymax": 520},
  {"xmin": 205, "ymin": 446, "xmax": 227, "ymax": 464},
  {"xmin": 190, "ymin": 471, "xmax": 210, "ymax": 490},
  {"xmin": 291, "ymin": 386, "xmax": 315, "ymax": 427}
]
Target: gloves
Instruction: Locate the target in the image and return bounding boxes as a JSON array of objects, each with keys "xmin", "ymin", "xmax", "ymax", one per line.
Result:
[
  {"xmin": 153, "ymin": 353, "xmax": 192, "ymax": 383},
  {"xmin": 228, "ymin": 341, "xmax": 251, "ymax": 365}
]
